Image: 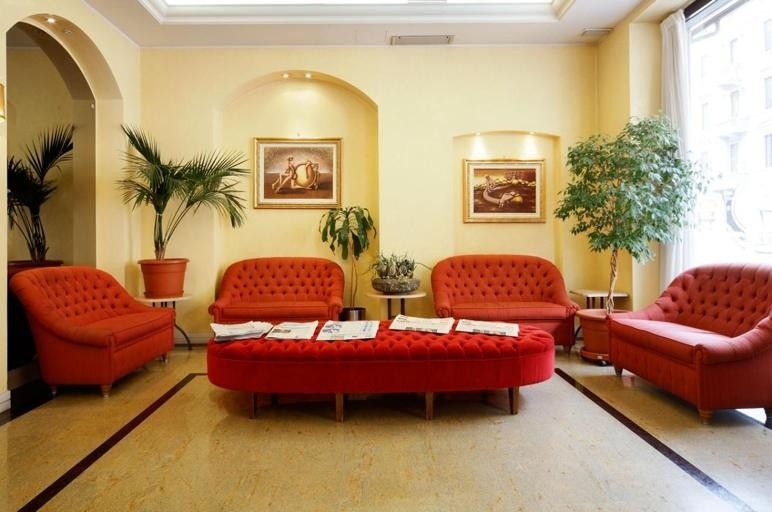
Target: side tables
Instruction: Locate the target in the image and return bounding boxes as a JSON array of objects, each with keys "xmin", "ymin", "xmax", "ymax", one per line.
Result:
[
  {"xmin": 137, "ymin": 296, "xmax": 193, "ymax": 350},
  {"xmin": 368, "ymin": 292, "xmax": 428, "ymax": 320},
  {"xmin": 569, "ymin": 289, "xmax": 628, "ymax": 310}
]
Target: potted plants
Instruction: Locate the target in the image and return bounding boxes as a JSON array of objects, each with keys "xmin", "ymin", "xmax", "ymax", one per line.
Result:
[
  {"xmin": 368, "ymin": 251, "xmax": 420, "ymax": 295},
  {"xmin": 0, "ymin": 121, "xmax": 77, "ymax": 272},
  {"xmin": 111, "ymin": 121, "xmax": 252, "ymax": 298},
  {"xmin": 318, "ymin": 206, "xmax": 377, "ymax": 321},
  {"xmin": 554, "ymin": 110, "xmax": 708, "ymax": 360}
]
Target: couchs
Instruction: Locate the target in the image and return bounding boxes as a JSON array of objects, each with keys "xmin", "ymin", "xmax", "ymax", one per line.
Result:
[
  {"xmin": 431, "ymin": 254, "xmax": 580, "ymax": 354},
  {"xmin": 608, "ymin": 262, "xmax": 772, "ymax": 426},
  {"xmin": 8, "ymin": 265, "xmax": 175, "ymax": 399},
  {"xmin": 208, "ymin": 256, "xmax": 345, "ymax": 321}
]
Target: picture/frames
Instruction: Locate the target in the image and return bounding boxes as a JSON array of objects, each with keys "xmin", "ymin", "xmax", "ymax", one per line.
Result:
[
  {"xmin": 253, "ymin": 136, "xmax": 343, "ymax": 210},
  {"xmin": 461, "ymin": 157, "xmax": 548, "ymax": 224}
]
